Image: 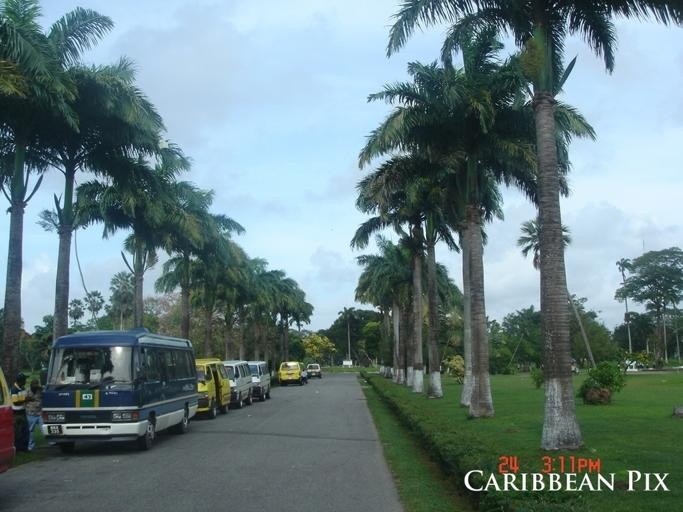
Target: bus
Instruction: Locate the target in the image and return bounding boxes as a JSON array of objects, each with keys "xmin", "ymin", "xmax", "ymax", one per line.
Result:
[{"xmin": 43, "ymin": 330, "xmax": 199, "ymax": 448}]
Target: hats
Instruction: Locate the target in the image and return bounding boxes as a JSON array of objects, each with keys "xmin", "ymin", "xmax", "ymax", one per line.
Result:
[{"xmin": 16, "ymin": 373, "xmax": 30, "ymax": 381}]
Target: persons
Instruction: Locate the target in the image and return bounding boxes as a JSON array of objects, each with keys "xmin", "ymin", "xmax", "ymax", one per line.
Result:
[
  {"xmin": 301, "ymin": 370, "xmax": 308, "ymax": 384},
  {"xmin": 9, "ymin": 372, "xmax": 35, "ymax": 452},
  {"xmin": 26, "ymin": 379, "xmax": 56, "ymax": 451}
]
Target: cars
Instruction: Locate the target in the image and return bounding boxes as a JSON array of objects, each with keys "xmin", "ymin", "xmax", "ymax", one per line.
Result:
[{"xmin": 571, "ymin": 363, "xmax": 579, "ymax": 374}]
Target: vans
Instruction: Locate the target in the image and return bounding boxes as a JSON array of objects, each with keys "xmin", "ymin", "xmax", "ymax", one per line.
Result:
[
  {"xmin": 307, "ymin": 364, "xmax": 321, "ymax": 378},
  {"xmin": 245, "ymin": 361, "xmax": 271, "ymax": 405},
  {"xmin": 0, "ymin": 365, "xmax": 16, "ymax": 475},
  {"xmin": 192, "ymin": 356, "xmax": 232, "ymax": 421},
  {"xmin": 278, "ymin": 361, "xmax": 307, "ymax": 387},
  {"xmin": 223, "ymin": 360, "xmax": 256, "ymax": 410}
]
